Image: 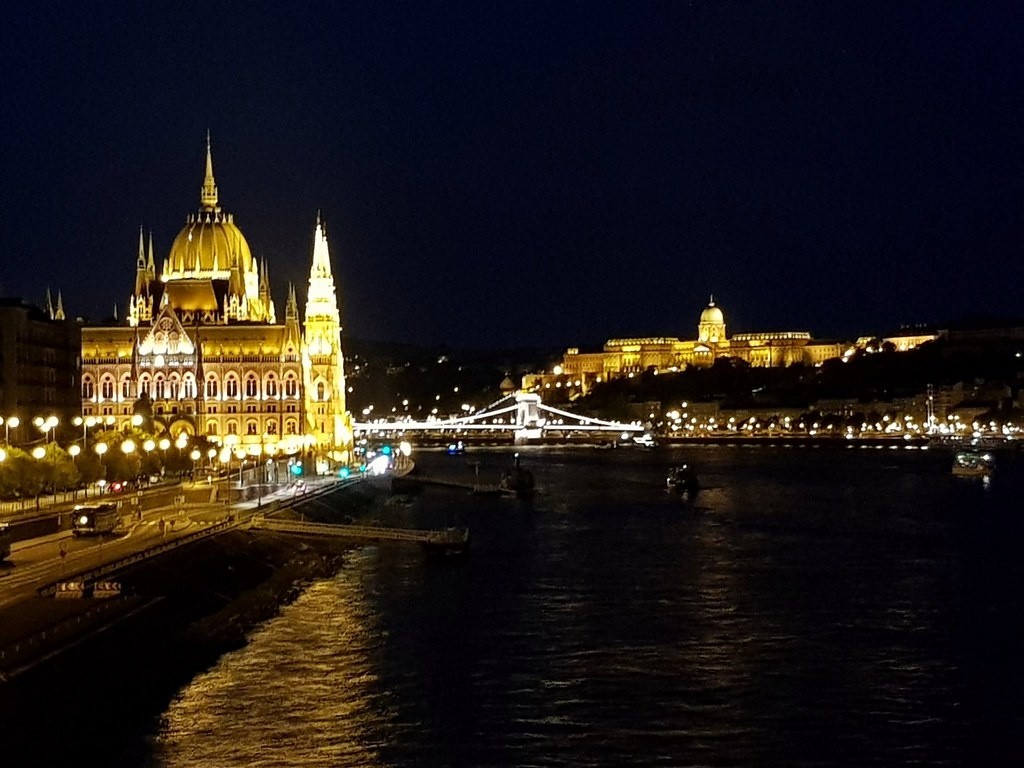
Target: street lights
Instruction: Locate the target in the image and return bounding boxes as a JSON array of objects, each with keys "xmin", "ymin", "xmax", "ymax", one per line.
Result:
[
  {"xmin": 68, "ymin": 444, "xmax": 81, "ymax": 462},
  {"xmin": 120, "ymin": 439, "xmax": 136, "ymax": 457},
  {"xmin": 143, "ymin": 439, "xmax": 155, "ymax": 456},
  {"xmin": 32, "ymin": 414, "xmax": 59, "ymax": 445},
  {"xmin": 131, "ymin": 413, "xmax": 145, "ymax": 431},
  {"xmin": 72, "ymin": 416, "xmax": 118, "ymax": 448},
  {"xmin": 93, "ymin": 441, "xmax": 108, "ymax": 461},
  {"xmin": 159, "ymin": 438, "xmax": 172, "ymax": 461},
  {"xmin": 0, "ymin": 417, "xmax": 20, "ymax": 447},
  {"xmin": 189, "ymin": 434, "xmax": 318, "ymax": 485},
  {"xmin": 176, "ymin": 435, "xmax": 192, "ymax": 458}
]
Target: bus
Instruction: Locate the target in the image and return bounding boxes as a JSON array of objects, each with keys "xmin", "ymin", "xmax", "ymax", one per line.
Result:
[{"xmin": 70, "ymin": 502, "xmax": 124, "ymax": 537}]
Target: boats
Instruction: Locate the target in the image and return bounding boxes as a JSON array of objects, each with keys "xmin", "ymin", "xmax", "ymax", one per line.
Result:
[
  {"xmin": 663, "ymin": 462, "xmax": 694, "ymax": 503},
  {"xmin": 952, "ymin": 450, "xmax": 996, "ymax": 486}
]
